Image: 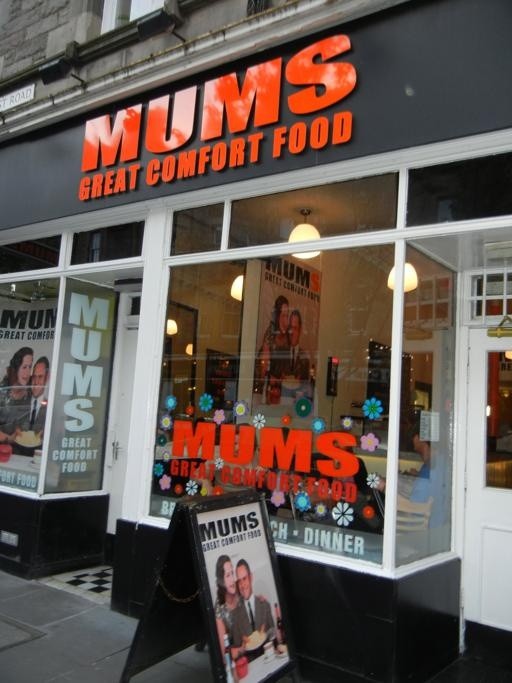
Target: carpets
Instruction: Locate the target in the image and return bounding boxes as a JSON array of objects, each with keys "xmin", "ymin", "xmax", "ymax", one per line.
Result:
[
  {"xmin": 248, "ymin": 602, "xmax": 256, "ymax": 632},
  {"xmin": 30, "ymin": 399, "xmax": 37, "ymax": 430},
  {"xmin": 291, "ymin": 347, "xmax": 295, "ymax": 370}
]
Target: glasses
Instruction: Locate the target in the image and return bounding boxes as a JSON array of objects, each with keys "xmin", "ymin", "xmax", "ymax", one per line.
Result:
[{"xmin": 286, "ymin": 207, "xmax": 322, "ymax": 261}]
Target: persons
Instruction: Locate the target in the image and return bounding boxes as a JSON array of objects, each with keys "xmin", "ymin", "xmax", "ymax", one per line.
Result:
[
  {"xmin": 253, "ymin": 294, "xmax": 314, "ymax": 402},
  {"xmin": 0, "ymin": 346, "xmax": 50, "ymax": 456},
  {"xmin": 375, "ymin": 420, "xmax": 451, "ymax": 554},
  {"xmin": 214, "ymin": 555, "xmax": 277, "ymax": 670}
]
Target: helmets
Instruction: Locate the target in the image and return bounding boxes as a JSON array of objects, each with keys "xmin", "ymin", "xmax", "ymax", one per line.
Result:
[
  {"xmin": 243, "ymin": 633, "xmax": 268, "ymax": 651},
  {"xmin": 13, "ymin": 440, "xmax": 40, "ymax": 448}
]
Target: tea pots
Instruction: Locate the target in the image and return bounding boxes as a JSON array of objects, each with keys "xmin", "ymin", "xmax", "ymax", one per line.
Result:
[{"xmin": 158, "ymin": 297, "xmax": 198, "ymax": 418}]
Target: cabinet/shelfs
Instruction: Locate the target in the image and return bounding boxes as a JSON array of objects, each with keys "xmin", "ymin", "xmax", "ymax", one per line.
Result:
[
  {"xmin": 275, "ymin": 602, "xmax": 285, "ymax": 645},
  {"xmin": 262, "ymin": 360, "xmax": 271, "ymax": 405},
  {"xmin": 225, "ymin": 631, "xmax": 237, "ymax": 682}
]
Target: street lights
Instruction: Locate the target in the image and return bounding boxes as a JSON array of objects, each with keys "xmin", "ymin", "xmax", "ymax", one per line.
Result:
[{"xmin": 253, "ymin": 358, "xmax": 264, "ymax": 395}]
